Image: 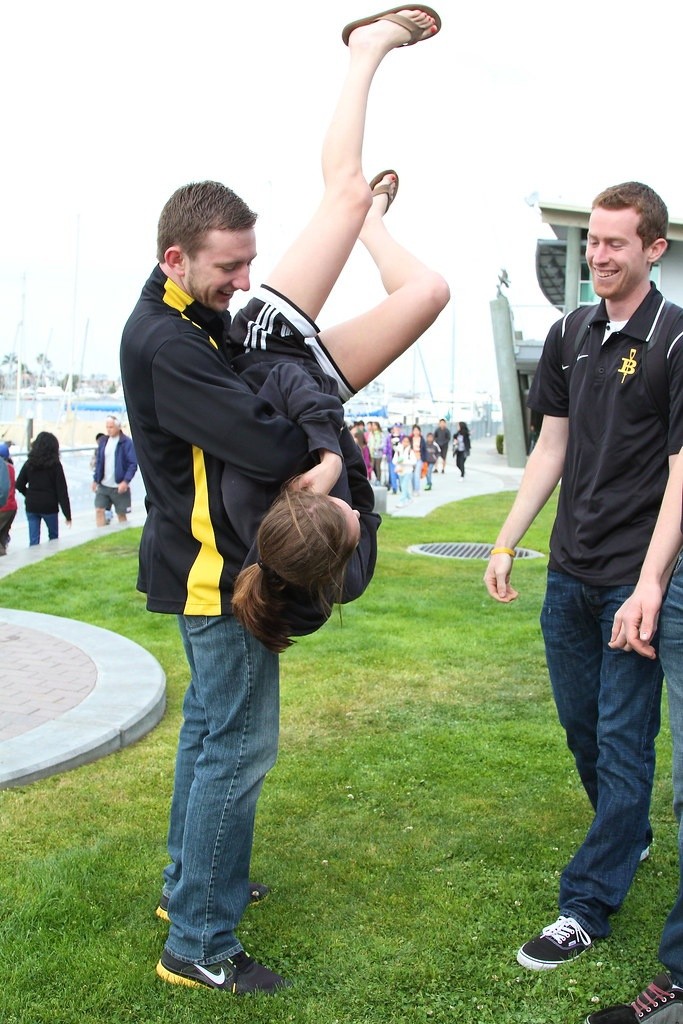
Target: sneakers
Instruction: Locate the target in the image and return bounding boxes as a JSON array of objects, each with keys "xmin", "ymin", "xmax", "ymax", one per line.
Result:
[
  {"xmin": 517, "ymin": 914, "xmax": 597, "ymax": 971},
  {"xmin": 640, "ymin": 846, "xmax": 649, "ymax": 862},
  {"xmin": 156, "ymin": 948, "xmax": 293, "ymax": 996},
  {"xmin": 156, "ymin": 884, "xmax": 271, "ymax": 922},
  {"xmin": 584, "ymin": 973, "xmax": 683, "ymax": 1024}
]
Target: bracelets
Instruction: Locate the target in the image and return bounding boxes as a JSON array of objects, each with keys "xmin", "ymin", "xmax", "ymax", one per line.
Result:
[{"xmin": 491, "ymin": 548, "xmax": 515, "ymax": 557}]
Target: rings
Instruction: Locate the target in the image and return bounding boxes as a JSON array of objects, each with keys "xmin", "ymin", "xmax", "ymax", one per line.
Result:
[{"xmin": 125, "ymin": 489, "xmax": 127, "ymax": 491}]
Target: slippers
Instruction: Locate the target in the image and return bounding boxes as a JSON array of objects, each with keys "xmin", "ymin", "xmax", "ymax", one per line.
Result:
[
  {"xmin": 342, "ymin": 5, "xmax": 442, "ymax": 48},
  {"xmin": 369, "ymin": 170, "xmax": 399, "ymax": 215}
]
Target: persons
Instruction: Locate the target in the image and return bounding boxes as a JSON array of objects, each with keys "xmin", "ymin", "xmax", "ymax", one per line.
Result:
[
  {"xmin": 0, "ymin": 440, "xmax": 17, "ymax": 555},
  {"xmin": 91, "ymin": 415, "xmax": 137, "ymax": 527},
  {"xmin": 121, "ymin": 181, "xmax": 352, "ymax": 993},
  {"xmin": 347, "ymin": 421, "xmax": 441, "ymax": 508},
  {"xmin": 483, "ymin": 182, "xmax": 683, "ymax": 1024},
  {"xmin": 433, "ymin": 419, "xmax": 450, "ymax": 473},
  {"xmin": 452, "ymin": 422, "xmax": 471, "ymax": 480},
  {"xmin": 15, "ymin": 431, "xmax": 71, "ymax": 547},
  {"xmin": 223, "ymin": 4, "xmax": 452, "ymax": 652}
]
